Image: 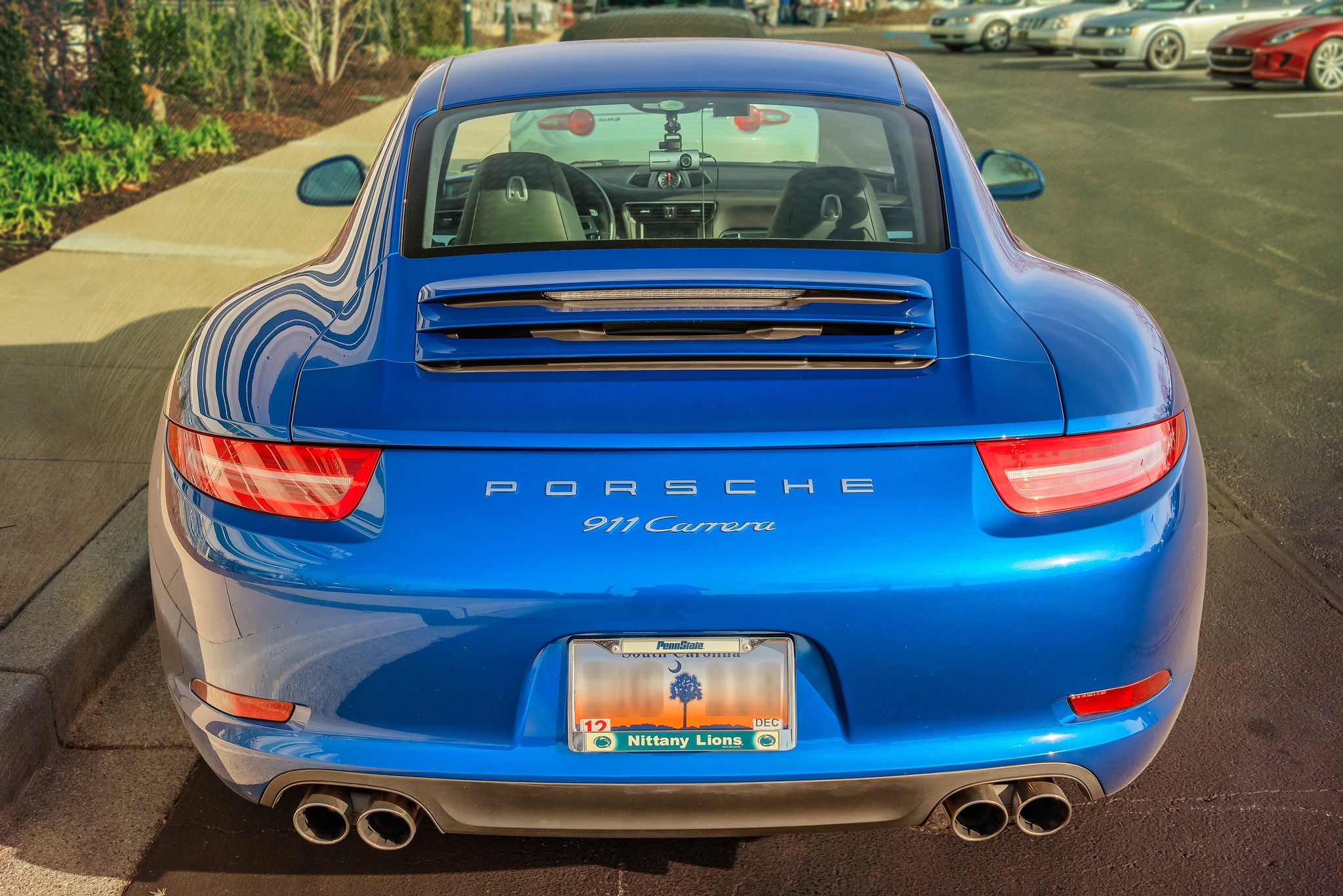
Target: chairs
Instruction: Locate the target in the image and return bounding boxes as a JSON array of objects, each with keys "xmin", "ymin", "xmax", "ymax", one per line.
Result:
[
  {"xmin": 765, "ymin": 166, "xmax": 890, "ymax": 241},
  {"xmin": 453, "ymin": 152, "xmax": 587, "ymax": 246}
]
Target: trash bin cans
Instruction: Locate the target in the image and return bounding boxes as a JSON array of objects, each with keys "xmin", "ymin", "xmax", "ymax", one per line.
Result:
[{"xmin": 812, "ymin": 4, "xmax": 826, "ymax": 28}]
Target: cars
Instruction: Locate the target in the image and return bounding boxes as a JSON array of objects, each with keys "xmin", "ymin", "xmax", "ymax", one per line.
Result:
[
  {"xmin": 925, "ymin": 0, "xmax": 1075, "ymax": 54},
  {"xmin": 1073, "ymin": 0, "xmax": 1320, "ymax": 71},
  {"xmin": 507, "ymin": 3, "xmax": 821, "ymax": 189},
  {"xmin": 1202, "ymin": 0, "xmax": 1343, "ymax": 93},
  {"xmin": 1019, "ymin": 0, "xmax": 1147, "ymax": 59}
]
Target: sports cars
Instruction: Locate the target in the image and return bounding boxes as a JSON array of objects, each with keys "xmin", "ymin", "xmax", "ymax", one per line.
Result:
[{"xmin": 145, "ymin": 38, "xmax": 1214, "ymax": 852}]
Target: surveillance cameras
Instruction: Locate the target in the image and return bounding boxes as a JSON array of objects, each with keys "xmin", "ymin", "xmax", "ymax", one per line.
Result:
[{"xmin": 649, "ymin": 149, "xmax": 700, "ymax": 172}]
